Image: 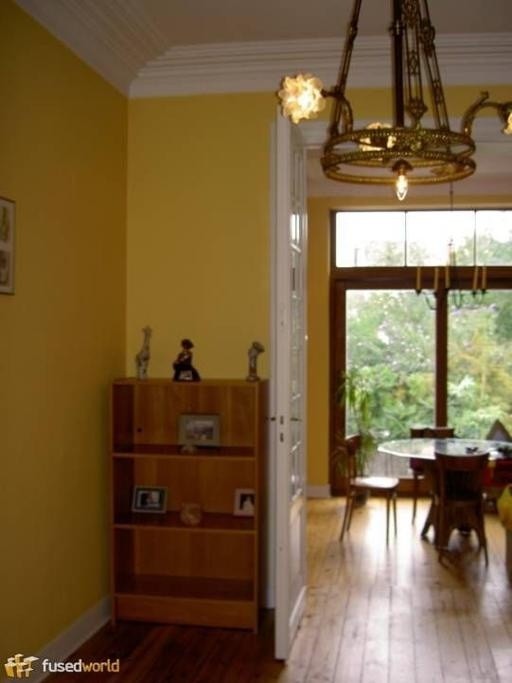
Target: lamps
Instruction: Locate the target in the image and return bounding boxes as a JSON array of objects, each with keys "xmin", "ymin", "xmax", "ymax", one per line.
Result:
[
  {"xmin": 275, "ymin": 0, "xmax": 511, "ymax": 203},
  {"xmin": 413, "ymin": 180, "xmax": 495, "ymax": 312}
]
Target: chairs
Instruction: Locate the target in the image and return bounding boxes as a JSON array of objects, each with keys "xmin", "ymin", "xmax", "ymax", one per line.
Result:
[{"xmin": 336, "ymin": 426, "xmax": 511, "ymax": 569}]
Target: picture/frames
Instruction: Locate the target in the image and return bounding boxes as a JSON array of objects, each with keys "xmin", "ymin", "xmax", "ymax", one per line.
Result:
[
  {"xmin": 231, "ymin": 486, "xmax": 256, "ymax": 517},
  {"xmin": 175, "ymin": 409, "xmax": 223, "ymax": 447},
  {"xmin": 0, "ymin": 193, "xmax": 19, "ymax": 296},
  {"xmin": 130, "ymin": 482, "xmax": 170, "ymax": 515}
]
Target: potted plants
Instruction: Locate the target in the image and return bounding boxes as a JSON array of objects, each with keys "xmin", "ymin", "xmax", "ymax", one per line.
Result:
[{"xmin": 329, "ymin": 362, "xmax": 379, "ymax": 511}]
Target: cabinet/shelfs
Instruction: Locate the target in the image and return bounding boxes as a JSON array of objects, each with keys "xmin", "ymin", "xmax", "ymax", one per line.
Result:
[{"xmin": 110, "ymin": 375, "xmax": 271, "ymax": 634}]
